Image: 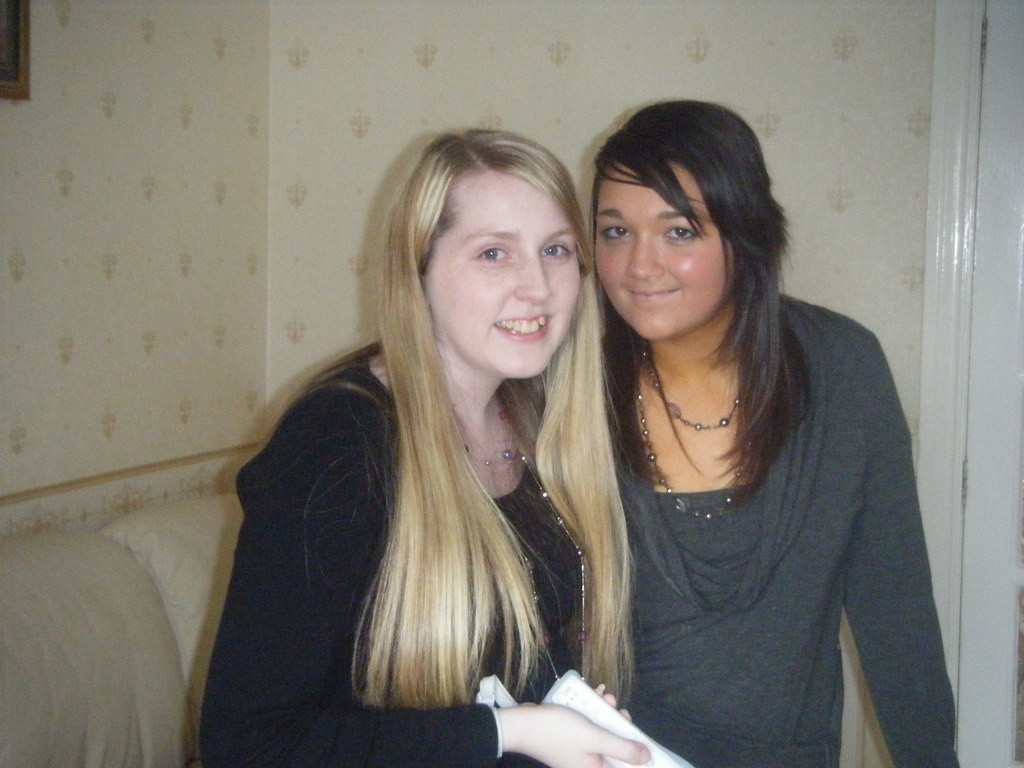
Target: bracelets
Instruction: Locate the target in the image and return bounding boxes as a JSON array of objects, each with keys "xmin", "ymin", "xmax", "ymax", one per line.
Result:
[{"xmin": 477, "ymin": 675, "xmax": 518, "ymax": 759}]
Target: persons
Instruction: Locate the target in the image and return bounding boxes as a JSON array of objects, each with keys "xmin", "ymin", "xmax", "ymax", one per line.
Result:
[
  {"xmin": 590, "ymin": 100, "xmax": 962, "ymax": 768},
  {"xmin": 199, "ymin": 129, "xmax": 651, "ymax": 768}
]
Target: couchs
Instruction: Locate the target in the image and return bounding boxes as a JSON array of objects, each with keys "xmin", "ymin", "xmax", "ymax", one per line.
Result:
[{"xmin": 0, "ymin": 494, "xmax": 863, "ymax": 768}]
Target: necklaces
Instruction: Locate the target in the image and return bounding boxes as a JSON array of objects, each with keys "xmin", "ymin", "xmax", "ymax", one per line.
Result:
[
  {"xmin": 466, "ymin": 411, "xmax": 586, "ymax": 682},
  {"xmin": 638, "ymin": 352, "xmax": 752, "ymax": 519}
]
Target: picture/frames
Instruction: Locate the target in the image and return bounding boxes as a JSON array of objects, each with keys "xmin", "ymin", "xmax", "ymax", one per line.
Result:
[{"xmin": 0, "ymin": 0, "xmax": 32, "ymax": 102}]
